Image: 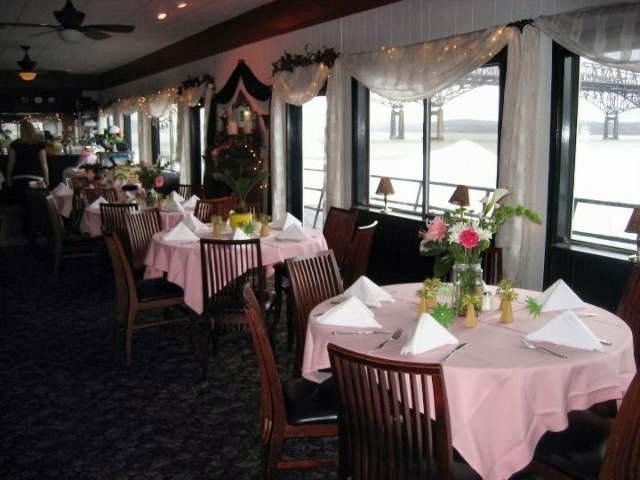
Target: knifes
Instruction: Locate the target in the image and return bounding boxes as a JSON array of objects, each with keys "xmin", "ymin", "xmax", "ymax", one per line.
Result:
[
  {"xmin": 444, "ymin": 342, "xmax": 469, "ymax": 362},
  {"xmin": 332, "ymin": 329, "xmax": 393, "ymax": 337}
]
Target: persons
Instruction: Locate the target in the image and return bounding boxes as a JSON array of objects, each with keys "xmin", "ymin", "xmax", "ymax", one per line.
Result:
[{"xmin": 6, "ymin": 122, "xmax": 49, "ymax": 239}]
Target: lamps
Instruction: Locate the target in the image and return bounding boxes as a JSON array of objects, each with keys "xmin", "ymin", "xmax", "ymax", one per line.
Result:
[
  {"xmin": 447, "ymin": 182, "xmax": 473, "ymax": 221},
  {"xmin": 60, "ymin": 28, "xmax": 84, "ymax": 44},
  {"xmin": 374, "ymin": 174, "xmax": 395, "ymax": 217},
  {"xmin": 16, "ymin": 45, "xmax": 39, "ymax": 82},
  {"xmin": 619, "ymin": 206, "xmax": 639, "ymax": 264}
]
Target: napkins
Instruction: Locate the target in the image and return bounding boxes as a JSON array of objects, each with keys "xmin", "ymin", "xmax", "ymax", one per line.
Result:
[
  {"xmin": 339, "ymin": 274, "xmax": 397, "ymax": 310},
  {"xmin": 523, "ymin": 310, "xmax": 608, "ymax": 356},
  {"xmin": 529, "ymin": 279, "xmax": 588, "ymax": 313},
  {"xmin": 316, "ymin": 296, "xmax": 385, "ymax": 334},
  {"xmin": 399, "ymin": 310, "xmax": 461, "ymax": 358}
]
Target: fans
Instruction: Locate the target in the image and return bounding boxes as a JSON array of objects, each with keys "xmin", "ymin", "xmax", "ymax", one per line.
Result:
[{"xmin": 0, "ymin": 1, "xmax": 134, "ymax": 41}]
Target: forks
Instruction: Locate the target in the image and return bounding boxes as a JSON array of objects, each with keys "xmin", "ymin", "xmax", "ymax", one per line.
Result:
[
  {"xmin": 519, "ymin": 336, "xmax": 568, "ymax": 360},
  {"xmin": 375, "ymin": 327, "xmax": 404, "ymax": 351}
]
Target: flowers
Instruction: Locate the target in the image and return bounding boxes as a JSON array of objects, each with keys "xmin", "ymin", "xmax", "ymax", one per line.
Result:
[{"xmin": 418, "ymin": 212, "xmax": 493, "ymax": 256}]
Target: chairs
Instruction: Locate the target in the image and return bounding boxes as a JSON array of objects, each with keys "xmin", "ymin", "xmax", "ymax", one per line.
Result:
[
  {"xmin": 326, "ymin": 341, "xmax": 457, "ymax": 480},
  {"xmin": 239, "ymin": 281, "xmax": 340, "ymax": 480},
  {"xmin": 24, "ymin": 153, "xmax": 378, "ymax": 365},
  {"xmin": 572, "ymin": 373, "xmax": 640, "ymax": 480},
  {"xmin": 614, "ymin": 266, "xmax": 639, "ymax": 361},
  {"xmin": 283, "ymin": 248, "xmax": 349, "ymax": 378},
  {"xmin": 427, "ymin": 243, "xmax": 507, "ymax": 285}
]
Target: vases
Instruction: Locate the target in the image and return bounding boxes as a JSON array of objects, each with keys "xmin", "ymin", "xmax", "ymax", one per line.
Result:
[{"xmin": 451, "ymin": 263, "xmax": 488, "ymax": 318}]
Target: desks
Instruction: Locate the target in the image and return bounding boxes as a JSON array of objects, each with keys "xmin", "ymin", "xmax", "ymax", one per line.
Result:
[{"xmin": 301, "ymin": 280, "xmax": 636, "ymax": 477}]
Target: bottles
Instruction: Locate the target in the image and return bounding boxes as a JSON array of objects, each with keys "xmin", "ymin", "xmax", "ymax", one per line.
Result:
[
  {"xmin": 482, "ymin": 291, "xmax": 494, "ymax": 312},
  {"xmin": 494, "ymin": 288, "xmax": 505, "ymax": 310}
]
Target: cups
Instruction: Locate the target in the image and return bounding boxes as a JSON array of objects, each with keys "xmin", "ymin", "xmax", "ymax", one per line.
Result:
[{"xmin": 159, "ymin": 198, "xmax": 168, "ymax": 212}]
